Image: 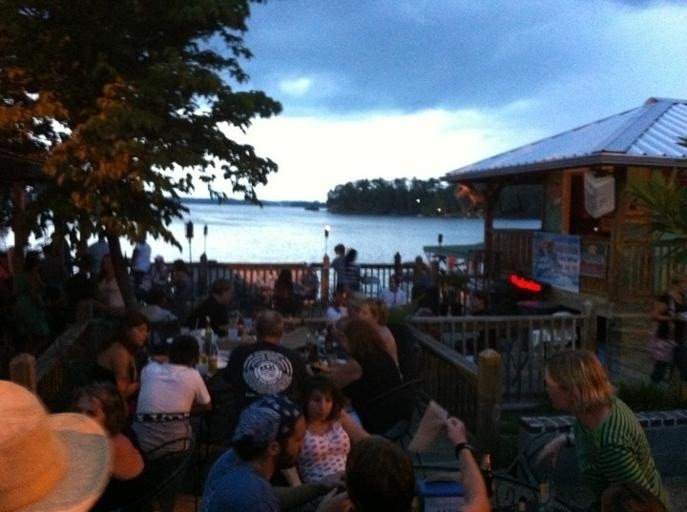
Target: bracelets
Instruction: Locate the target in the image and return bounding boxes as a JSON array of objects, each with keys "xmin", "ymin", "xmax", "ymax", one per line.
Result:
[{"xmin": 455, "ymin": 443, "xmax": 475, "ymax": 460}]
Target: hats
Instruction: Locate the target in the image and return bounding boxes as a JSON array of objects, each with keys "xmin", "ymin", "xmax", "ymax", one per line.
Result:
[
  {"xmin": 231, "ymin": 392, "xmax": 304, "ymax": 464},
  {"xmin": 1, "ymin": 381, "xmax": 110, "ymax": 511}
]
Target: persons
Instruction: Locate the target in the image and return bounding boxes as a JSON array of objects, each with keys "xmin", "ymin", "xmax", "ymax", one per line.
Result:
[
  {"xmin": 348, "ymin": 403, "xmax": 492, "ymax": 511},
  {"xmin": 92, "ymin": 313, "xmax": 151, "ymax": 400},
  {"xmin": 456, "ymin": 292, "xmax": 496, "ymax": 352},
  {"xmin": 224, "ymin": 309, "xmax": 309, "ymax": 435},
  {"xmin": 650, "ymin": 274, "xmax": 687, "ymax": 405},
  {"xmin": 301, "ymin": 267, "xmax": 318, "ymax": 300},
  {"xmin": 412, "ymin": 255, "xmax": 431, "ymax": 292},
  {"xmin": 203, "ymin": 397, "xmax": 346, "ymax": 512},
  {"xmin": 132, "ymin": 336, "xmax": 211, "ymax": 461},
  {"xmin": 196, "ymin": 280, "xmax": 232, "ymax": 327},
  {"xmin": 379, "ymin": 275, "xmax": 407, "ymax": 308},
  {"xmin": 326, "ymin": 290, "xmax": 350, "ymax": 323},
  {"xmin": 273, "ymin": 270, "xmax": 301, "ymax": 316},
  {"xmin": 314, "ymin": 317, "xmax": 401, "ymax": 435},
  {"xmin": 535, "ymin": 351, "xmax": 673, "ymax": 511},
  {"xmin": 345, "ymin": 249, "xmax": 359, "ymax": 307},
  {"xmin": 0, "ymin": 231, "xmax": 194, "ymax": 336},
  {"xmin": 358, "ymin": 299, "xmax": 401, "ymax": 377},
  {"xmin": 333, "ymin": 245, "xmax": 345, "ymax": 295},
  {"xmin": 73, "ymin": 384, "xmax": 155, "ymax": 511},
  {"xmin": 282, "ymin": 374, "xmax": 372, "ymax": 510}
]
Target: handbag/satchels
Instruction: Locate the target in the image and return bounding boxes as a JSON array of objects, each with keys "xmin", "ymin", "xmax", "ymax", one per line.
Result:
[
  {"xmin": 652, "ymin": 337, "xmax": 677, "ymax": 364},
  {"xmin": 648, "ymin": 338, "xmax": 678, "ymax": 362}
]
